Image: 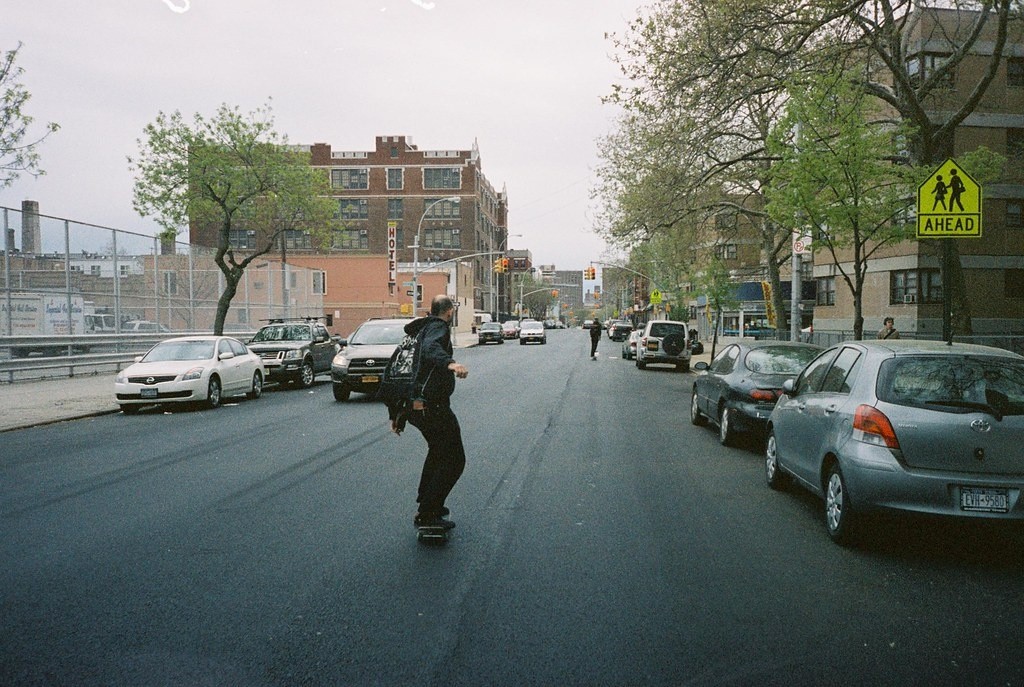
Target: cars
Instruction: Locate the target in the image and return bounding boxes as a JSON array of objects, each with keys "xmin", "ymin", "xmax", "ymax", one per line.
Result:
[
  {"xmin": 764, "ymin": 340, "xmax": 1023, "ymax": 551},
  {"xmin": 622, "ymin": 329, "xmax": 645, "ymax": 359},
  {"xmin": 114, "ymin": 336, "xmax": 265, "ymax": 414},
  {"xmin": 636, "ymin": 320, "xmax": 693, "ymax": 371},
  {"xmin": 331, "ymin": 316, "xmax": 424, "ymax": 401},
  {"xmin": 478, "ymin": 322, "xmax": 503, "ymax": 344},
  {"xmin": 582, "ymin": 318, "xmax": 635, "ymax": 341},
  {"xmin": 690, "ymin": 338, "xmax": 829, "ymax": 446},
  {"xmin": 90, "ymin": 314, "xmax": 253, "ymax": 330},
  {"xmin": 503, "ymin": 319, "xmax": 566, "ymax": 340},
  {"xmin": 519, "ymin": 322, "xmax": 546, "ymax": 344}
]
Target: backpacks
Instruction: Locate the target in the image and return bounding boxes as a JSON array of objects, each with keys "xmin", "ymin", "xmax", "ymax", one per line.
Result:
[
  {"xmin": 382, "ymin": 319, "xmax": 451, "ymax": 396},
  {"xmin": 590, "ymin": 324, "xmax": 599, "ymax": 336}
]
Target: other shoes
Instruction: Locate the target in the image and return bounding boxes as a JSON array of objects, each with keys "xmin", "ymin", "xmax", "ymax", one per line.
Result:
[
  {"xmin": 417, "ymin": 507, "xmax": 450, "ymax": 515},
  {"xmin": 414, "ymin": 512, "xmax": 455, "ymax": 530}
]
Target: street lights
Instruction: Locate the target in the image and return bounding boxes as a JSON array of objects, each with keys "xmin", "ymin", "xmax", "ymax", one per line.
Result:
[
  {"xmin": 407, "ymin": 197, "xmax": 461, "ymax": 316},
  {"xmin": 517, "ymin": 266, "xmax": 539, "ymax": 319},
  {"xmin": 489, "ymin": 234, "xmax": 522, "ymax": 313}
]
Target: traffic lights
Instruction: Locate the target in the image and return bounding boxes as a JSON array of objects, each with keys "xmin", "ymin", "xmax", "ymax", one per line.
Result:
[
  {"xmin": 584, "ymin": 267, "xmax": 596, "ymax": 280},
  {"xmin": 552, "ymin": 291, "xmax": 559, "ymax": 298},
  {"xmin": 495, "ymin": 259, "xmax": 509, "ymax": 274}
]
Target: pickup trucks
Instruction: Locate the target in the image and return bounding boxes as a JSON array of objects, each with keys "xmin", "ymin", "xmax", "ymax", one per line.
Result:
[{"xmin": 244, "ymin": 317, "xmax": 343, "ymax": 388}]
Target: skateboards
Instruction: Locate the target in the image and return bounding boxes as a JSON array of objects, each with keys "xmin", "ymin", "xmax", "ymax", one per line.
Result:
[{"xmin": 415, "ymin": 506, "xmax": 449, "ymax": 543}]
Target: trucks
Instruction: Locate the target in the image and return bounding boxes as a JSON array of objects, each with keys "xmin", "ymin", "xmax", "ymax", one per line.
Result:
[{"xmin": 0, "ymin": 292, "xmax": 96, "ymax": 359}]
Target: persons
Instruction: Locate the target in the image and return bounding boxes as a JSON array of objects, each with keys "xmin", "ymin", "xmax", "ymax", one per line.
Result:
[
  {"xmin": 589, "ymin": 317, "xmax": 602, "ymax": 358},
  {"xmin": 806, "ymin": 323, "xmax": 812, "ymax": 343},
  {"xmin": 391, "ymin": 293, "xmax": 469, "ymax": 530},
  {"xmin": 753, "ymin": 322, "xmax": 760, "ymax": 340},
  {"xmin": 877, "ymin": 316, "xmax": 900, "ymax": 340}
]
